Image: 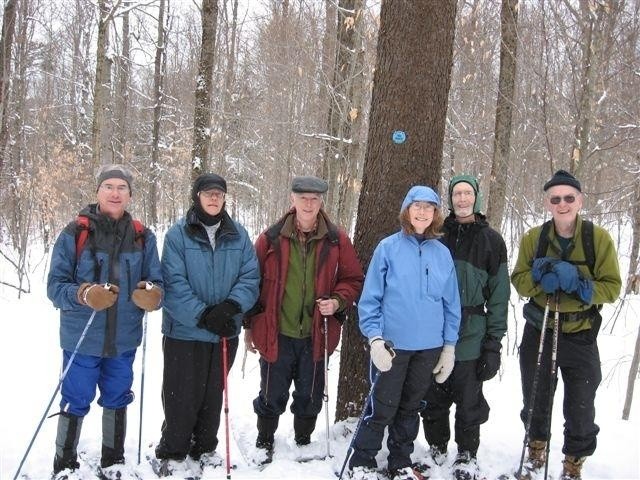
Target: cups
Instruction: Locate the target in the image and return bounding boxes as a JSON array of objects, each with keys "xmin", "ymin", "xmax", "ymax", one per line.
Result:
[
  {"xmin": 99, "ymin": 182, "xmax": 130, "ymax": 194},
  {"xmin": 200, "ymin": 188, "xmax": 226, "ymax": 201},
  {"xmin": 408, "ymin": 199, "xmax": 436, "ymax": 214},
  {"xmin": 546, "ymin": 194, "xmax": 578, "ymax": 206}
]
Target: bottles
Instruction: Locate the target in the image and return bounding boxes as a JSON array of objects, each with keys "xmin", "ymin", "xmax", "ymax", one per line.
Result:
[
  {"xmin": 96, "ymin": 164, "xmax": 135, "ymax": 199},
  {"xmin": 191, "ymin": 173, "xmax": 228, "ymax": 193},
  {"xmin": 292, "ymin": 175, "xmax": 330, "ymax": 194},
  {"xmin": 543, "ymin": 169, "xmax": 583, "ymax": 193},
  {"xmin": 446, "ymin": 174, "xmax": 482, "ymax": 216}
]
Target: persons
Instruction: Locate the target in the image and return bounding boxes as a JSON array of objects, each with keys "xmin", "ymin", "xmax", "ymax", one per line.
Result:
[
  {"xmin": 510, "ymin": 169, "xmax": 623, "ymax": 480},
  {"xmin": 45, "ymin": 163, "xmax": 165, "ymax": 480},
  {"xmin": 151, "ymin": 171, "xmax": 261, "ymax": 480},
  {"xmin": 242, "ymin": 175, "xmax": 366, "ymax": 469},
  {"xmin": 346, "ymin": 184, "xmax": 463, "ymax": 480},
  {"xmin": 419, "ymin": 173, "xmax": 510, "ymax": 480}
]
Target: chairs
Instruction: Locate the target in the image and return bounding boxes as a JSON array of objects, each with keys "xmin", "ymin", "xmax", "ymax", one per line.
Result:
[
  {"xmin": 147, "ymin": 444, "xmax": 190, "ymax": 479},
  {"xmin": 193, "ymin": 446, "xmax": 237, "ymax": 476},
  {"xmin": 451, "ymin": 449, "xmax": 477, "ymax": 480},
  {"xmin": 389, "ymin": 458, "xmax": 423, "ymax": 479},
  {"xmin": 351, "ymin": 464, "xmax": 377, "ymax": 479},
  {"xmin": 428, "ymin": 438, "xmax": 448, "ymax": 468}
]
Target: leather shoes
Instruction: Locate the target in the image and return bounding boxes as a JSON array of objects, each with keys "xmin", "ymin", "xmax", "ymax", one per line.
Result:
[
  {"xmin": 77, "ymin": 280, "xmax": 121, "ymax": 313},
  {"xmin": 367, "ymin": 335, "xmax": 397, "ymax": 374},
  {"xmin": 431, "ymin": 342, "xmax": 458, "ymax": 385},
  {"xmin": 554, "ymin": 256, "xmax": 594, "ymax": 307},
  {"xmin": 476, "ymin": 339, "xmax": 502, "ymax": 382},
  {"xmin": 205, "ymin": 300, "xmax": 242, "ymax": 329},
  {"xmin": 530, "ymin": 255, "xmax": 560, "ymax": 294},
  {"xmin": 197, "ymin": 305, "xmax": 237, "ymax": 338},
  {"xmin": 129, "ymin": 280, "xmax": 164, "ymax": 314}
]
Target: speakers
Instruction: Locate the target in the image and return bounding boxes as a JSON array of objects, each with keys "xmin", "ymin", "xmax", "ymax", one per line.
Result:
[
  {"xmin": 293, "ymin": 412, "xmax": 318, "ymax": 463},
  {"xmin": 252, "ymin": 414, "xmax": 280, "ymax": 467},
  {"xmin": 512, "ymin": 439, "xmax": 550, "ymax": 480},
  {"xmin": 560, "ymin": 453, "xmax": 588, "ymax": 479},
  {"xmin": 96, "ymin": 405, "xmax": 127, "ymax": 479},
  {"xmin": 52, "ymin": 410, "xmax": 85, "ymax": 480}
]
